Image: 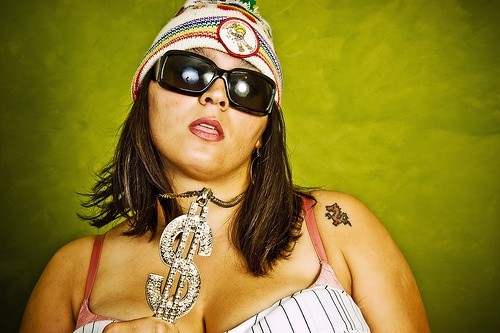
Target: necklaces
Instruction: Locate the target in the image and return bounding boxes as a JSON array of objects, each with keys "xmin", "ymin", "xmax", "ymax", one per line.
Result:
[{"xmin": 146, "ymin": 186, "xmax": 246, "ymax": 319}]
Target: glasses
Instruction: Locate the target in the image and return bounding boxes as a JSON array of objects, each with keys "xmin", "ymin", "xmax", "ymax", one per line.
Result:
[{"xmin": 148, "ymin": 49, "xmax": 278, "ymax": 116}]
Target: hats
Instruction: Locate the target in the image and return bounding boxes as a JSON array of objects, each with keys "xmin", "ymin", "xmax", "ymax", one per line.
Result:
[{"xmin": 131, "ymin": 0, "xmax": 282, "ymax": 107}]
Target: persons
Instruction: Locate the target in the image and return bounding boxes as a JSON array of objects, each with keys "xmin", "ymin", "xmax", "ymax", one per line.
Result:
[{"xmin": 20, "ymin": 1, "xmax": 429, "ymax": 332}]
